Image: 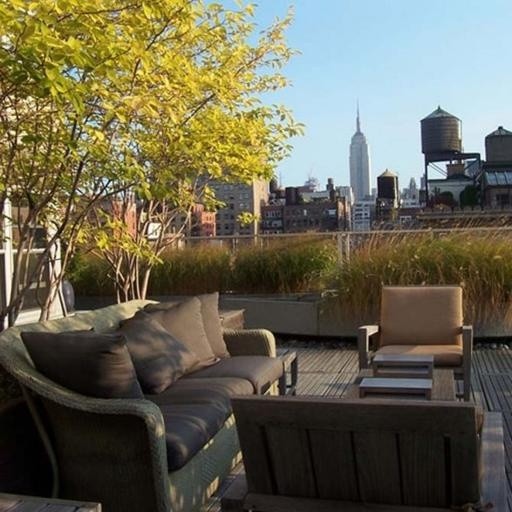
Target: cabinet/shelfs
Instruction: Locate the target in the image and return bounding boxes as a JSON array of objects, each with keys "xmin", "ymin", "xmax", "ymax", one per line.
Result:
[{"xmin": 217, "ymin": 307, "xmax": 246, "ymax": 330}]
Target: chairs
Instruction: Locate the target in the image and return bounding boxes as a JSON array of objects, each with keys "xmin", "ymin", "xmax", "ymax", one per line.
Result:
[
  {"xmin": 355, "ymin": 286, "xmax": 474, "ymax": 402},
  {"xmin": 220, "ymin": 391, "xmax": 508, "ymax": 512}
]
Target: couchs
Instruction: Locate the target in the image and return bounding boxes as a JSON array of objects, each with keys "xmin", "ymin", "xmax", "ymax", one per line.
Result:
[{"xmin": 0, "ymin": 298, "xmax": 284, "ymax": 512}]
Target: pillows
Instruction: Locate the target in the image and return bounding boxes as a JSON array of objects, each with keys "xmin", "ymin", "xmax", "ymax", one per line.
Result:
[{"xmin": 20, "ymin": 283, "xmax": 231, "ymax": 399}]
